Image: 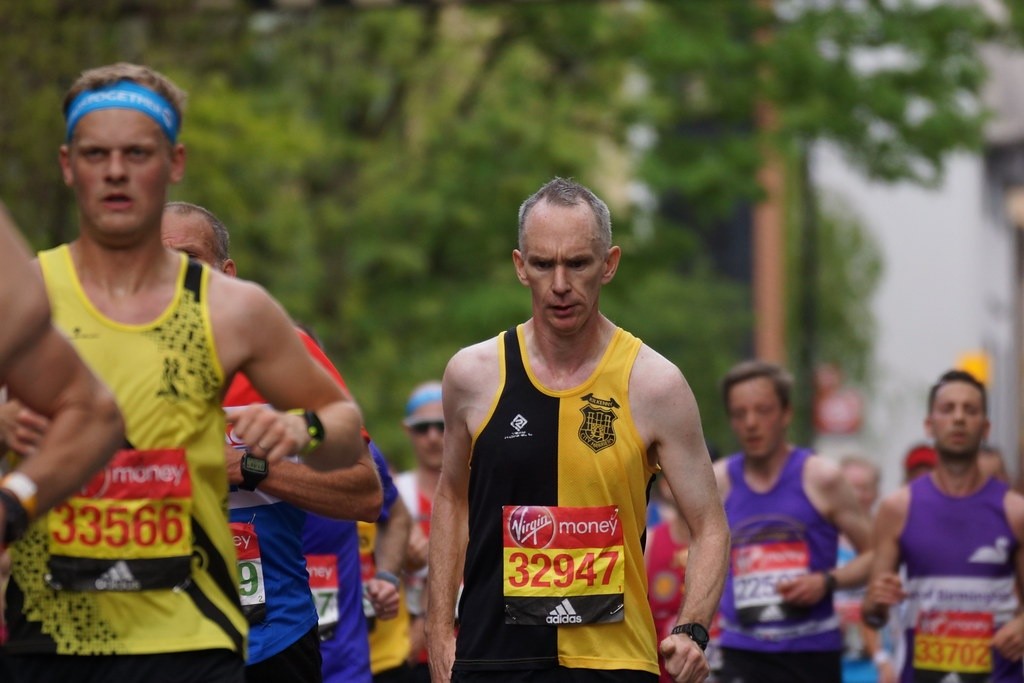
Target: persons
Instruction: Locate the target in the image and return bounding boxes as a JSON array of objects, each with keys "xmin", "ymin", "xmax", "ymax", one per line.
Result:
[
  {"xmin": 163, "ymin": 200, "xmax": 427, "ymax": 683},
  {"xmin": 861, "ymin": 370, "xmax": 1024, "ymax": 683},
  {"xmin": 0, "ymin": 205, "xmax": 125, "ymax": 683},
  {"xmin": 687, "ymin": 360, "xmax": 883, "ymax": 683},
  {"xmin": 0, "ymin": 60, "xmax": 363, "ymax": 683},
  {"xmin": 424, "ymin": 175, "xmax": 732, "ymax": 683},
  {"xmin": 391, "ymin": 380, "xmax": 466, "ymax": 683}
]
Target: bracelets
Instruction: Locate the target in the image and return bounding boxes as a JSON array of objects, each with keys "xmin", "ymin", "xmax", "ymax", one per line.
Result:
[
  {"xmin": 376, "ymin": 571, "xmax": 399, "ymax": 592},
  {"xmin": 286, "ymin": 408, "xmax": 324, "ymax": 458},
  {"xmin": 2, "ymin": 471, "xmax": 41, "ymax": 520},
  {"xmin": 872, "ymin": 649, "xmax": 889, "ymax": 665}
]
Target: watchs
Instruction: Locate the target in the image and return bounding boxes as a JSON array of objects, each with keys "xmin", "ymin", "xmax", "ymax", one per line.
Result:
[
  {"xmin": 825, "ymin": 569, "xmax": 838, "ymax": 592},
  {"xmin": 670, "ymin": 623, "xmax": 710, "ymax": 651},
  {"xmin": 241, "ymin": 449, "xmax": 268, "ymax": 492}
]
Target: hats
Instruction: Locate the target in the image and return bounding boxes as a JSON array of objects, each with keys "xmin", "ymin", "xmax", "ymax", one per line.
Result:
[{"xmin": 903, "ymin": 442, "xmax": 941, "ymax": 471}]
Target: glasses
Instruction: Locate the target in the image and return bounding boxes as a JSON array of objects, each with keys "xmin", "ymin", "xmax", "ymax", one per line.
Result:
[{"xmin": 410, "ymin": 419, "xmax": 445, "ymax": 435}]
